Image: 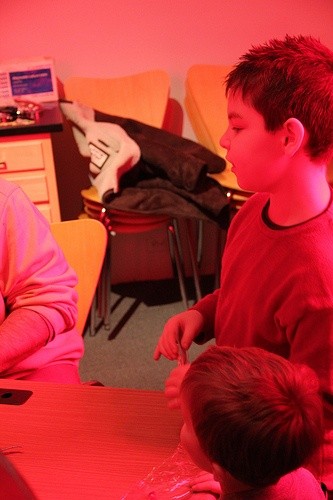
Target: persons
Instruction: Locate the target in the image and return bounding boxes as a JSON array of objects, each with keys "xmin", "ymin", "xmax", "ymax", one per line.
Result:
[
  {"xmin": 152, "ymin": 35, "xmax": 333, "ymax": 398},
  {"xmin": 0, "ymin": 180, "xmax": 79, "ymax": 383},
  {"xmin": 171, "ymin": 345, "xmax": 329, "ymax": 500}
]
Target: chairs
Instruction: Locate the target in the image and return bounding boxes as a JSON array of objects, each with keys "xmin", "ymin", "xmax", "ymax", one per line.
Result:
[{"xmin": 51, "ymin": 62, "xmax": 255, "ymax": 339}]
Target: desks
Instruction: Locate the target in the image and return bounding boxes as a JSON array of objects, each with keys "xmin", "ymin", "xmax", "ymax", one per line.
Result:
[{"xmin": 0, "ymin": 379, "xmax": 217, "ymax": 500}]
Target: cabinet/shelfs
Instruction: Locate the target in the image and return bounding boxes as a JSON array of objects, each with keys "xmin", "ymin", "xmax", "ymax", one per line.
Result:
[{"xmin": 0, "ymin": 105, "xmax": 92, "ymax": 224}]
